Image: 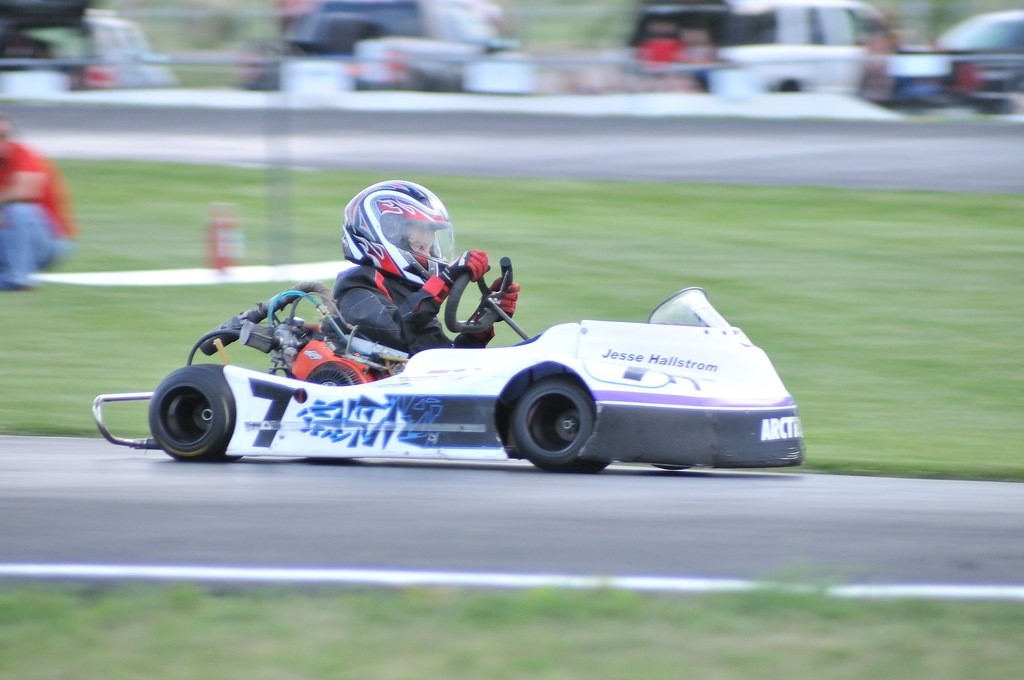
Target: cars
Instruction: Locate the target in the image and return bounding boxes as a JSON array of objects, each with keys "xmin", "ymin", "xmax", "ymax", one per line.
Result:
[
  {"xmin": 627, "ymin": 0, "xmax": 887, "ymax": 96},
  {"xmin": 285, "ymin": 3, "xmax": 499, "ymax": 92},
  {"xmin": 1, "ymin": 0, "xmax": 178, "ymax": 89},
  {"xmin": 859, "ymin": 7, "xmax": 1024, "ymax": 116}
]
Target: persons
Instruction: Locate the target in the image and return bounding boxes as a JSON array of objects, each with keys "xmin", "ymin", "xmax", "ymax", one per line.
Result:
[
  {"xmin": 0, "ymin": 116, "xmax": 81, "ymax": 290},
  {"xmin": 320, "ymin": 179, "xmax": 518, "ymax": 354}
]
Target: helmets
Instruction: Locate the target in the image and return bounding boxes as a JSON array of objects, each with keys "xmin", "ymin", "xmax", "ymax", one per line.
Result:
[{"xmin": 341, "ymin": 180, "xmax": 456, "ymax": 285}]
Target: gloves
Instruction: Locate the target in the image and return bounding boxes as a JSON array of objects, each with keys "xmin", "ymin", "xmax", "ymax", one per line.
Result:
[
  {"xmin": 488, "ymin": 276, "xmax": 521, "ymax": 322},
  {"xmin": 438, "ymin": 250, "xmax": 488, "ymax": 287}
]
